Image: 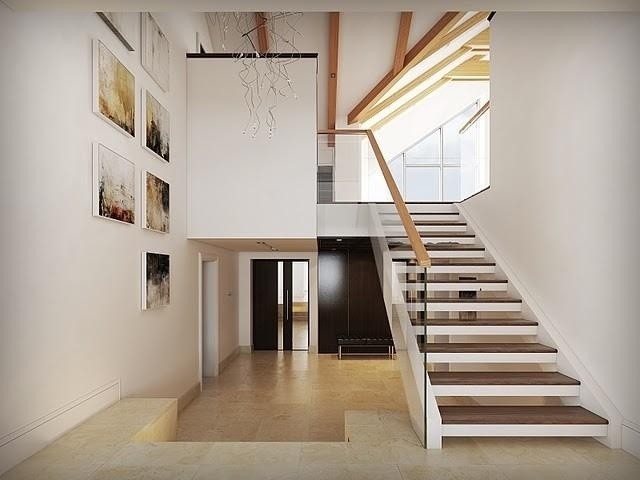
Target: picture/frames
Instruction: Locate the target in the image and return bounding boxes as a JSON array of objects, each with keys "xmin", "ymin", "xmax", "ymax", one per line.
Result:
[{"xmin": 91, "ymin": 11, "xmax": 172, "ymax": 311}]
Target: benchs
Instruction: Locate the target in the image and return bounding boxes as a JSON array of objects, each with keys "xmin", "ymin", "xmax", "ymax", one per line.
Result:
[{"xmin": 335, "ymin": 334, "xmax": 395, "ymax": 361}]
